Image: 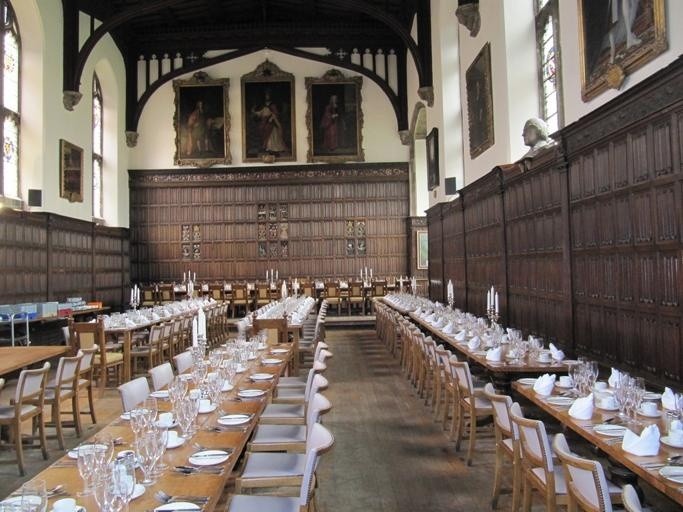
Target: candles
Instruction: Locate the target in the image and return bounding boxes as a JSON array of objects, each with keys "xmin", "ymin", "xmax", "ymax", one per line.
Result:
[
  {"xmin": 275, "ymin": 268, "xmax": 279, "ymax": 280},
  {"xmin": 360, "ymin": 268, "xmax": 363, "ymax": 279},
  {"xmin": 365, "ymin": 266, "xmax": 368, "ymax": 280},
  {"xmin": 266, "ymin": 270, "xmax": 269, "ymax": 280},
  {"xmin": 370, "ymin": 267, "xmax": 373, "ymax": 279},
  {"xmin": 183, "ymin": 272, "xmax": 187, "ymax": 280},
  {"xmin": 270, "ymin": 268, "xmax": 274, "ymax": 278},
  {"xmin": 188, "ymin": 268, "xmax": 191, "ymax": 280},
  {"xmin": 193, "ymin": 272, "xmax": 197, "ymax": 282}
]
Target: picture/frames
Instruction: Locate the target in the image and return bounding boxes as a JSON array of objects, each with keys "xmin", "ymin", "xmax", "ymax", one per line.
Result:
[
  {"xmin": 577, "ymin": 1, "xmax": 668, "ymax": 103},
  {"xmin": 416, "ymin": 231, "xmax": 428, "ymax": 270},
  {"xmin": 426, "ymin": 128, "xmax": 439, "ymax": 191},
  {"xmin": 172, "ymin": 72, "xmax": 232, "ymax": 165},
  {"xmin": 60, "ymin": 138, "xmax": 84, "ymax": 202},
  {"xmin": 305, "ymin": 69, "xmax": 368, "ymax": 164},
  {"xmin": 241, "ymin": 60, "xmax": 298, "ymax": 163},
  {"xmin": 465, "ymin": 42, "xmax": 495, "ymax": 158}
]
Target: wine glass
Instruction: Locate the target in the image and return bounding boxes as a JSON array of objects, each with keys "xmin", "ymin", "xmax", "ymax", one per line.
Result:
[
  {"xmin": 384, "ymin": 289, "xmax": 682, "ymax": 490},
  {"xmin": 0, "ymin": 327, "xmax": 268, "ymax": 511},
  {"xmin": 101, "ymin": 286, "xmax": 307, "ymax": 324}
]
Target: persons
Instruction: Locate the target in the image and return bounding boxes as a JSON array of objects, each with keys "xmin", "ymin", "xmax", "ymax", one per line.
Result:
[
  {"xmin": 318, "ymin": 94, "xmax": 348, "ymax": 150},
  {"xmin": 249, "ymin": 87, "xmax": 290, "ymax": 157},
  {"xmin": 186, "ymin": 98, "xmax": 217, "ymax": 155}
]
